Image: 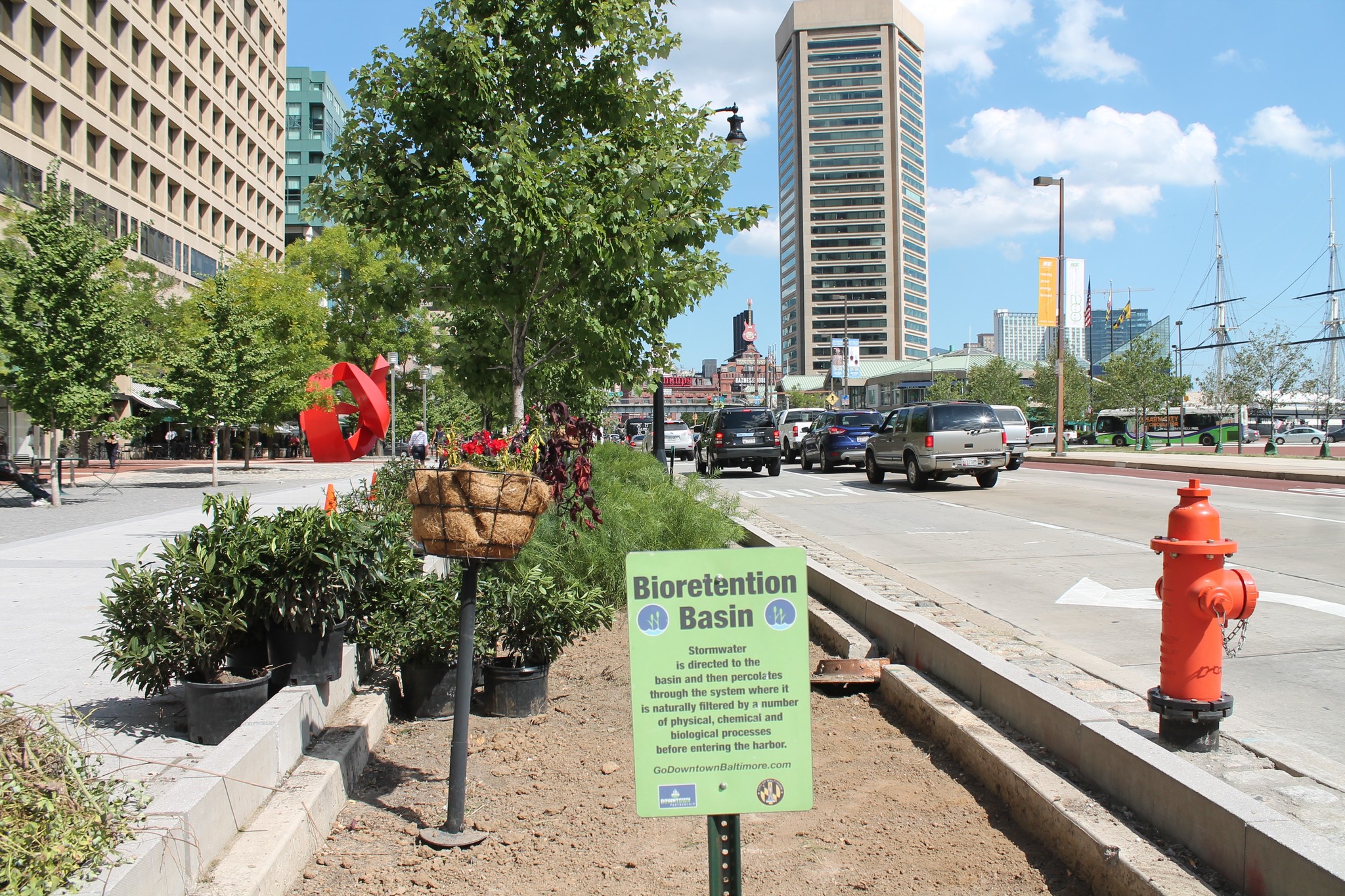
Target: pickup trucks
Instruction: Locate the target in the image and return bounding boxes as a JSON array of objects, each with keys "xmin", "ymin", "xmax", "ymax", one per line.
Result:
[
  {"xmin": 773, "ymin": 407, "xmax": 828, "ymax": 463},
  {"xmin": 1030, "ymin": 426, "xmax": 1078, "ymax": 446}
]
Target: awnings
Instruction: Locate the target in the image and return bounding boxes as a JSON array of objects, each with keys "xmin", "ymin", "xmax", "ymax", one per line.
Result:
[
  {"xmin": 1019, "ymin": 379, "xmax": 1035, "ymax": 386},
  {"xmin": 897, "ymin": 380, "xmax": 957, "ymax": 389},
  {"xmin": 1025, "ymin": 414, "xmax": 1041, "ymax": 421},
  {"xmin": 130, "ymin": 383, "xmax": 182, "ymax": 410}
]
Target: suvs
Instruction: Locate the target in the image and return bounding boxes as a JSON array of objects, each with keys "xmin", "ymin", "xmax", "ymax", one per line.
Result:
[
  {"xmin": 693, "ymin": 406, "xmax": 781, "ymax": 475},
  {"xmin": 865, "ymin": 399, "xmax": 1012, "ymax": 490},
  {"xmin": 800, "ymin": 408, "xmax": 885, "ymax": 474},
  {"xmin": 988, "ymin": 405, "xmax": 1032, "ymax": 470}
]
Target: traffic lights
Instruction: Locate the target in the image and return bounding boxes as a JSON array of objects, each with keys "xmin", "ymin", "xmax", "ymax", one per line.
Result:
[
  {"xmin": 707, "ymin": 396, "xmax": 711, "ymax": 407},
  {"xmin": 695, "ymin": 414, "xmax": 697, "ymax": 419},
  {"xmin": 692, "ymin": 415, "xmax": 694, "ymax": 420}
]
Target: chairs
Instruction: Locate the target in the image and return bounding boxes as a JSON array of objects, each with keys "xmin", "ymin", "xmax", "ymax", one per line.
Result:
[
  {"xmin": 93, "ymin": 451, "xmax": 124, "ymax": 496},
  {"xmin": 97, "ymin": 441, "xmax": 215, "ymax": 459},
  {"xmin": 14, "ymin": 454, "xmax": 46, "ymax": 491}
]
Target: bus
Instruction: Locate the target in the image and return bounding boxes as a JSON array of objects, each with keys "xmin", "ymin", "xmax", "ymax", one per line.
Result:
[{"xmin": 1096, "ymin": 404, "xmax": 1249, "ymax": 447}]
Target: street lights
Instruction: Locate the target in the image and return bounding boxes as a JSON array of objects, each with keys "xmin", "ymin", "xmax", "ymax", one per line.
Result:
[
  {"xmin": 831, "ymin": 294, "xmax": 848, "ymax": 407},
  {"xmin": 926, "ymin": 359, "xmax": 934, "ymax": 387},
  {"xmin": 1172, "ymin": 345, "xmax": 1178, "ymax": 392},
  {"xmin": 1175, "ymin": 321, "xmax": 1183, "ymax": 394},
  {"xmin": 1033, "ymin": 176, "xmax": 1069, "ymax": 455},
  {"xmin": 388, "ymin": 352, "xmax": 432, "ymax": 460}
]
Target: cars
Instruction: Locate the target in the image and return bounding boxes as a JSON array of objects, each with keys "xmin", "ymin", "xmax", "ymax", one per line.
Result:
[
  {"xmin": 1079, "ymin": 432, "xmax": 1098, "ymax": 445},
  {"xmin": 1272, "ymin": 427, "xmax": 1329, "ymax": 445},
  {"xmin": 1328, "ymin": 427, "xmax": 1345, "ymax": 443},
  {"xmin": 604, "ymin": 416, "xmax": 703, "ymax": 460},
  {"xmin": 368, "ymin": 442, "xmax": 413, "ymax": 457},
  {"xmin": 1242, "ymin": 428, "xmax": 1261, "ymax": 443}
]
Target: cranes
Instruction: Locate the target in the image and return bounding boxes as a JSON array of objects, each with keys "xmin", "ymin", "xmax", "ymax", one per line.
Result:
[{"xmin": 1084, "ymin": 288, "xmax": 1154, "ymax": 295}]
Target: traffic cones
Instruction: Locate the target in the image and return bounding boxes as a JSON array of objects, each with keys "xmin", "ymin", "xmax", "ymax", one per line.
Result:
[
  {"xmin": 365, "ymin": 472, "xmax": 378, "ymax": 501},
  {"xmin": 324, "ymin": 483, "xmax": 339, "ymax": 516}
]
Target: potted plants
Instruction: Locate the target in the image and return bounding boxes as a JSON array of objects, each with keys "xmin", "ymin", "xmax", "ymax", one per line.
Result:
[
  {"xmin": 365, "ymin": 566, "xmax": 480, "ymax": 722},
  {"xmin": 191, "ymin": 427, "xmax": 208, "ymax": 459},
  {"xmin": 218, "ymin": 443, "xmax": 233, "ymax": 459},
  {"xmin": 484, "ymin": 563, "xmax": 614, "ymax": 717},
  {"xmin": 82, "ymin": 491, "xmax": 400, "ymax": 745},
  {"xmin": 237, "ymin": 436, "xmax": 256, "ymax": 458}
]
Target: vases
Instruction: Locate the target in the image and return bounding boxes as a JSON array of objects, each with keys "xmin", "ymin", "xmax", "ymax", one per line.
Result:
[
  {"xmin": 263, "ymin": 448, "xmax": 268, "ymax": 457},
  {"xmin": 306, "ymin": 448, "xmax": 311, "ymax": 457},
  {"xmin": 275, "ymin": 448, "xmax": 287, "ymax": 457}
]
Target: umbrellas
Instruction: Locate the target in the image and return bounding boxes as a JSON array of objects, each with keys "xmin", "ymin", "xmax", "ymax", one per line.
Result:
[
  {"xmin": 280, "ymin": 423, "xmax": 295, "ymax": 430},
  {"xmin": 176, "ymin": 414, "xmax": 292, "ymax": 433},
  {"xmin": 1044, "ymin": 421, "xmax": 1101, "ymax": 426}
]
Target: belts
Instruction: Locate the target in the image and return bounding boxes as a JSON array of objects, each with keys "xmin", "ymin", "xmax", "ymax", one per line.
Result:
[{"xmin": 414, "ymin": 445, "xmax": 423, "ymax": 446}]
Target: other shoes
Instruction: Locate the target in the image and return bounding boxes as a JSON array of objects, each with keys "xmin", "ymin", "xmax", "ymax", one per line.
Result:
[
  {"xmin": 110, "ymin": 467, "xmax": 114, "ymax": 469},
  {"xmin": 36, "ymin": 498, "xmax": 51, "ymax": 505},
  {"xmin": 30, "ymin": 501, "xmax": 45, "ymax": 508},
  {"xmin": 435, "ymin": 459, "xmax": 437, "ymax": 462}
]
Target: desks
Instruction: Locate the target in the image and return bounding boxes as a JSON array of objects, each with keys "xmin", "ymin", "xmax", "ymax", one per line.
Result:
[{"xmin": 33, "ymin": 457, "xmax": 86, "ymax": 495}]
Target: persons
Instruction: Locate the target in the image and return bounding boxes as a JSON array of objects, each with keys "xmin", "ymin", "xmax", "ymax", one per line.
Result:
[
  {"xmin": 408, "ymin": 421, "xmax": 446, "ymax": 468},
  {"xmin": 102, "ymin": 416, "xmax": 120, "ymax": 469},
  {"xmin": 250, "ymin": 440, "xmax": 262, "ymax": 448},
  {"xmin": 284, "ymin": 433, "xmax": 300, "ymax": 458},
  {"xmin": 1322, "ymin": 422, "xmax": 1329, "ymax": 432},
  {"xmin": 349, "ymin": 432, "xmax": 352, "ymax": 438},
  {"xmin": 383, "ymin": 441, "xmax": 387, "ymax": 452},
  {"xmin": 165, "ymin": 427, "xmax": 178, "ymax": 458},
  {"xmin": 0, "ymin": 427, "xmax": 52, "ymax": 506},
  {"xmin": 1285, "ymin": 423, "xmax": 1291, "ymax": 434}
]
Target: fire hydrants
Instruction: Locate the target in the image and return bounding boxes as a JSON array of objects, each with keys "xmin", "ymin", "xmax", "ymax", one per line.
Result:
[{"xmin": 1147, "ymin": 478, "xmax": 1261, "ymax": 751}]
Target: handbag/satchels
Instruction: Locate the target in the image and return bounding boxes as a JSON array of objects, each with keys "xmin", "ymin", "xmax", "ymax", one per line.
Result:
[
  {"xmin": 410, "ymin": 449, "xmax": 412, "ymax": 455},
  {"xmin": 297, "ymin": 442, "xmax": 301, "ymax": 446},
  {"xmin": 28, "ymin": 434, "xmax": 34, "ymax": 446}
]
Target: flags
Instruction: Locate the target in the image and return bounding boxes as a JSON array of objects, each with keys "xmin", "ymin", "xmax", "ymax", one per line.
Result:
[
  {"xmin": 1085, "ymin": 281, "xmax": 1131, "ymax": 329},
  {"xmin": 1084, "ymin": 406, "xmax": 1093, "ymax": 419},
  {"xmin": 1273, "ymin": 419, "xmax": 1283, "ymax": 429},
  {"xmin": 768, "ymin": 349, "xmax": 776, "ymax": 369},
  {"xmin": 1305, "ymin": 422, "xmax": 1308, "ymax": 427}
]
